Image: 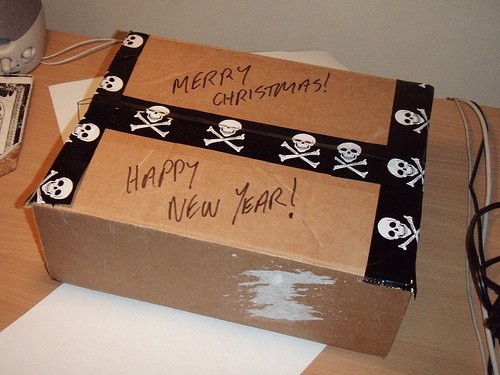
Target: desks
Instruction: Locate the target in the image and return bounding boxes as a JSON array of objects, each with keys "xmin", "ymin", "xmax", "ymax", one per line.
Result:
[{"xmin": 0, "ymin": 29, "xmax": 500, "ymax": 375}]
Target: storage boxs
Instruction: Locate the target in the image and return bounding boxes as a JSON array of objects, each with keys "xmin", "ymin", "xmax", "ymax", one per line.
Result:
[{"xmin": 34, "ymin": 31, "xmax": 438, "ymax": 358}]
